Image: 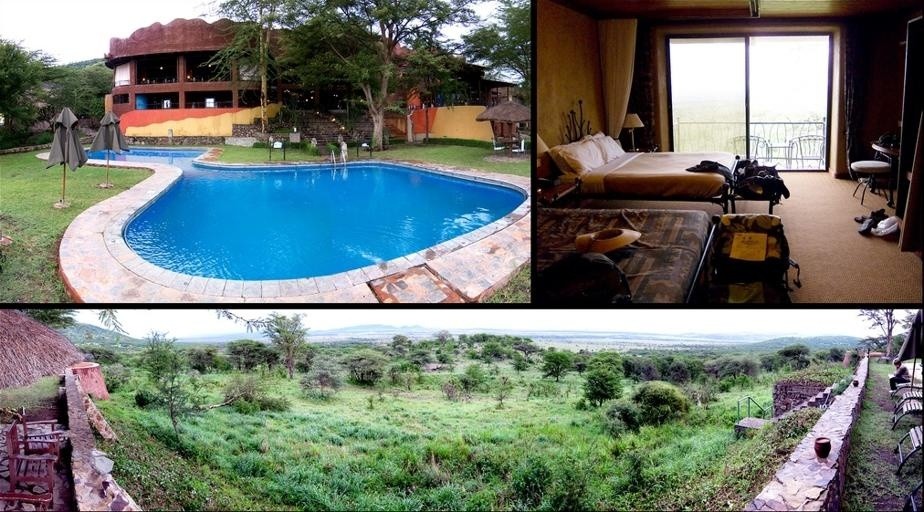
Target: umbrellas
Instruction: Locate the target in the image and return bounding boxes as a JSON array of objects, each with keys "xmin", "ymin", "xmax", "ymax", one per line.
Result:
[
  {"xmin": 88, "ymin": 110, "xmax": 131, "ymax": 186},
  {"xmin": 44, "ymin": 107, "xmax": 87, "ymax": 204},
  {"xmin": 898, "ymin": 308, "xmax": 923, "ymax": 395}
]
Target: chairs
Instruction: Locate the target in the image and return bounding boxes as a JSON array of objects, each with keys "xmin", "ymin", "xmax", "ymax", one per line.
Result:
[
  {"xmin": 493, "ymin": 138, "xmax": 505, "ymax": 158},
  {"xmin": 512, "ymin": 139, "xmax": 525, "ymax": 161},
  {"xmin": 792, "ymin": 135, "xmax": 825, "ymax": 166},
  {"xmin": 735, "ymin": 135, "xmax": 770, "ymax": 166},
  {"xmin": 891, "ymin": 384, "xmax": 924, "ymax": 512}
]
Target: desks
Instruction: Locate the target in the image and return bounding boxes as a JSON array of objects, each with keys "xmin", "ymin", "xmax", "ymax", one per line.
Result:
[{"xmin": 768, "ymin": 143, "xmax": 793, "ymax": 167}]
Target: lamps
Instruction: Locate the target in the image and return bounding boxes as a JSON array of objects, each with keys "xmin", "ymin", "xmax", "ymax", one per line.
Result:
[
  {"xmin": 537, "ymin": 133, "xmax": 549, "ymax": 168},
  {"xmin": 623, "ymin": 113, "xmax": 645, "ymax": 151}
]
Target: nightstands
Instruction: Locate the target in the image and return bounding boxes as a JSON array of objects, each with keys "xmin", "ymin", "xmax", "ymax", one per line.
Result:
[
  {"xmin": 541, "ymin": 177, "xmax": 584, "ymax": 206},
  {"xmin": 629, "ymin": 147, "xmax": 650, "ymax": 152}
]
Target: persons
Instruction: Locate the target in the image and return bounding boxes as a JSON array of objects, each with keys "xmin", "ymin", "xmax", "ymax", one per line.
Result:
[{"xmin": 888, "ymin": 358, "xmax": 912, "ymax": 392}]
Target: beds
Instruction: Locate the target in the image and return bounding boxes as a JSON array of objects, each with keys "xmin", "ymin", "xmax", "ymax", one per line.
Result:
[
  {"xmin": 548, "ymin": 131, "xmax": 741, "ymax": 212},
  {"xmin": 531, "ymin": 205, "xmax": 720, "ymax": 309}
]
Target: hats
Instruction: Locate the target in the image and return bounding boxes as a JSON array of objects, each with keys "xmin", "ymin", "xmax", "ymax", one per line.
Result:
[{"xmin": 573, "ymin": 225, "xmax": 641, "ymax": 255}]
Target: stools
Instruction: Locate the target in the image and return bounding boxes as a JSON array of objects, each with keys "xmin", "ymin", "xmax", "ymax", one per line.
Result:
[
  {"xmin": 850, "ymin": 158, "xmax": 890, "ymax": 205},
  {"xmin": 871, "ymin": 144, "xmax": 899, "ymax": 156}
]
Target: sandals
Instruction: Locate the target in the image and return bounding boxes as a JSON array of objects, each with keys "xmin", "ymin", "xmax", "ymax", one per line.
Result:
[{"xmin": 853, "ymin": 208, "xmax": 890, "ymax": 235}]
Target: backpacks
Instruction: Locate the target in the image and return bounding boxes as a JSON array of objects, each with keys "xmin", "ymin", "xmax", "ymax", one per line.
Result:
[{"xmin": 537, "ymin": 252, "xmax": 631, "ymax": 307}]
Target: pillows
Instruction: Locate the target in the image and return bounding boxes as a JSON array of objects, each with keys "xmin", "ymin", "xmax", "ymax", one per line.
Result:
[
  {"xmin": 596, "ymin": 135, "xmax": 627, "ymax": 162},
  {"xmin": 559, "ymin": 141, "xmax": 601, "ymax": 177},
  {"xmin": 594, "ymin": 131, "xmax": 605, "ymax": 139},
  {"xmin": 548, "ymin": 135, "xmax": 593, "ymax": 176}
]
